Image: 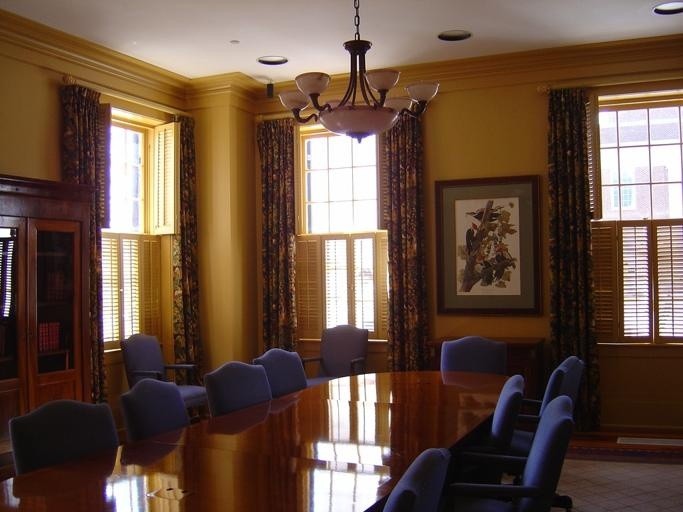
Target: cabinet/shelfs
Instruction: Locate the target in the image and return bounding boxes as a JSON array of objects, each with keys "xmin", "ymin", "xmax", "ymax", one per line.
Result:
[{"xmin": 0, "ymin": 173, "xmax": 95, "ymax": 456}]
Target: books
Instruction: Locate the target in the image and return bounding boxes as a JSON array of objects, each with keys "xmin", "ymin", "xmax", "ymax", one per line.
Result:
[{"xmin": 38, "ymin": 322, "xmax": 60, "ymax": 351}]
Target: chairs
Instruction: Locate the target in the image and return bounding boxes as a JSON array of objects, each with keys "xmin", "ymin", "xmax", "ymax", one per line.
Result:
[
  {"xmin": 302, "ymin": 324, "xmax": 370, "ymax": 388},
  {"xmin": 118, "ymin": 379, "xmax": 189, "ymax": 441},
  {"xmin": 119, "ymin": 333, "xmax": 207, "ymax": 418},
  {"xmin": 382, "ymin": 336, "xmax": 586, "ymax": 512},
  {"xmin": 203, "ymin": 361, "xmax": 272, "ymax": 416},
  {"xmin": 252, "ymin": 348, "xmax": 308, "ymax": 398},
  {"xmin": 9, "ymin": 399, "xmax": 119, "ymax": 475}
]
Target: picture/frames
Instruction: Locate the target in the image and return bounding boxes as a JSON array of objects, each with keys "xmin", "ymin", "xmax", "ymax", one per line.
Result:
[{"xmin": 434, "ymin": 174, "xmax": 545, "ymax": 316}]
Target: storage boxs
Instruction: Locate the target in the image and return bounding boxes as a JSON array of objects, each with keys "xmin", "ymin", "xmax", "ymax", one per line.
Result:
[
  {"xmin": 38, "ymin": 322, "xmax": 61, "ymax": 354},
  {"xmin": 37, "ymin": 269, "xmax": 65, "ymax": 302}
]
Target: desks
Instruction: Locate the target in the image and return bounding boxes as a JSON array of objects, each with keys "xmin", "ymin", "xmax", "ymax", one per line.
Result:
[{"xmin": 1, "ymin": 370, "xmax": 512, "ymax": 512}]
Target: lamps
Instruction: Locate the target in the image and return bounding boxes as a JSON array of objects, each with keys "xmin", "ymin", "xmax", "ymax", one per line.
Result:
[{"xmin": 275, "ymin": 0, "xmax": 439, "ymax": 142}]
